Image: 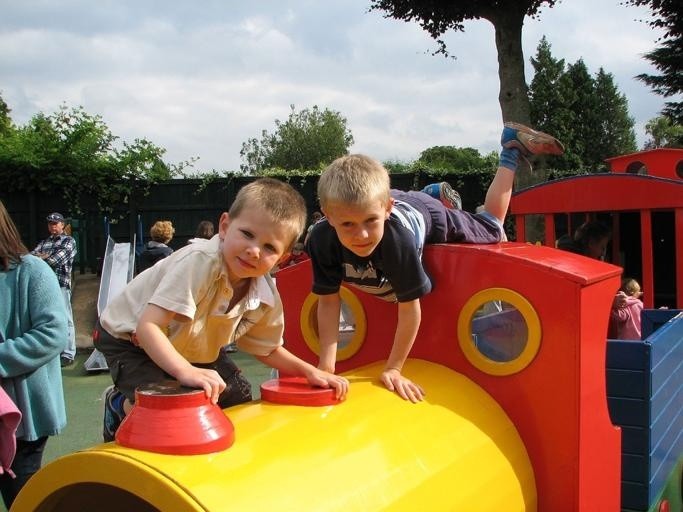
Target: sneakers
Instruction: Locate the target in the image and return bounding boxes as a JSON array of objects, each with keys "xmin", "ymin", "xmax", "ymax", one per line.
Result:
[
  {"xmin": 501, "ymin": 122, "xmax": 565, "ymax": 155},
  {"xmin": 60, "ymin": 357, "xmax": 73, "ymax": 367},
  {"xmin": 424, "ymin": 182, "xmax": 462, "ymax": 209},
  {"xmin": 102, "ymin": 385, "xmax": 123, "ymax": 441}
]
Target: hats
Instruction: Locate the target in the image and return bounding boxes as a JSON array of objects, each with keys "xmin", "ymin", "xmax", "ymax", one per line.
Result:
[{"xmin": 47, "ymin": 213, "xmax": 63, "ymax": 223}]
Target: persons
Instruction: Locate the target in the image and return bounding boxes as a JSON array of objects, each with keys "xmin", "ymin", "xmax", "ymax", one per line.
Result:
[
  {"xmin": 0, "ymin": 200, "xmax": 73, "ymax": 511},
  {"xmin": 555, "ymin": 223, "xmax": 626, "ymax": 309},
  {"xmin": 138, "ymin": 206, "xmax": 324, "ymax": 272},
  {"xmin": 27, "ymin": 212, "xmax": 80, "ymax": 368},
  {"xmin": 608, "ymin": 279, "xmax": 645, "ymax": 339},
  {"xmin": 95, "ymin": 177, "xmax": 350, "ymax": 442},
  {"xmin": 307, "ymin": 119, "xmax": 565, "ymax": 403}
]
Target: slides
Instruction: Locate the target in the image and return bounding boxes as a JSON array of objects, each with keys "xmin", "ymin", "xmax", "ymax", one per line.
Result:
[{"xmin": 84, "ymin": 215, "xmax": 142, "ymax": 371}]
